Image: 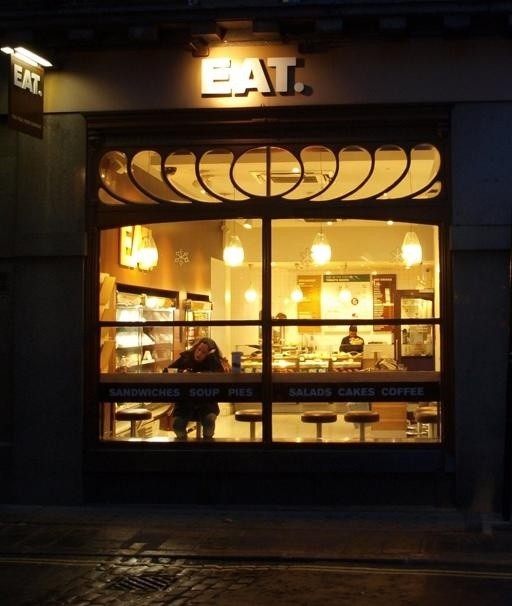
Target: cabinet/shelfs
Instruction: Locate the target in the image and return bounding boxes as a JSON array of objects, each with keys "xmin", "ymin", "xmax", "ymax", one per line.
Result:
[{"xmin": 115, "ymin": 305, "xmax": 211, "ymax": 413}]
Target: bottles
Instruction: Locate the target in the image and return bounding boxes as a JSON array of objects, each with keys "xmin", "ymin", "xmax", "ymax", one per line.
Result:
[
  {"xmin": 403, "ymin": 329, "xmax": 407, "ymax": 343},
  {"xmin": 121, "ymin": 356, "xmax": 126, "ymax": 367}
]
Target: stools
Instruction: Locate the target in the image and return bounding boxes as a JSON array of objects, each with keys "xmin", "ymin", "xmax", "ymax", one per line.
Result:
[
  {"xmin": 414, "ymin": 401, "xmax": 441, "ymax": 440},
  {"xmin": 301, "ymin": 410, "xmax": 336, "ymax": 440},
  {"xmin": 235, "ymin": 409, "xmax": 262, "ymax": 439},
  {"xmin": 344, "ymin": 410, "xmax": 381, "ymax": 441},
  {"xmin": 116, "ymin": 409, "xmax": 151, "ymax": 438}
]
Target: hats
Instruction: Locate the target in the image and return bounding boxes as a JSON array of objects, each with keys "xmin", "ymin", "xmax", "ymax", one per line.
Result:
[{"xmin": 350, "ymin": 326, "xmax": 357, "ymax": 331}]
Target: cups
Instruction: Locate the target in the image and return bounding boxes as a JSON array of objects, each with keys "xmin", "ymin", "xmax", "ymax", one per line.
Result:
[{"xmin": 168, "ymin": 368, "xmax": 177, "ymax": 373}]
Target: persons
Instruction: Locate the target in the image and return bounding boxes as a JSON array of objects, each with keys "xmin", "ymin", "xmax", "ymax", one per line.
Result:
[
  {"xmin": 162, "ymin": 337, "xmax": 222, "ymax": 439},
  {"xmin": 339, "ymin": 326, "xmax": 364, "ymax": 353}
]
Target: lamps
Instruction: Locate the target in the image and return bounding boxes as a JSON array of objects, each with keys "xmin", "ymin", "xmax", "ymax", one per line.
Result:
[
  {"xmin": 222, "ymin": 219, "xmax": 245, "ymax": 266},
  {"xmin": 402, "ymin": 223, "xmax": 422, "ymax": 265},
  {"xmin": 310, "ymin": 219, "xmax": 331, "ymax": 265}
]
